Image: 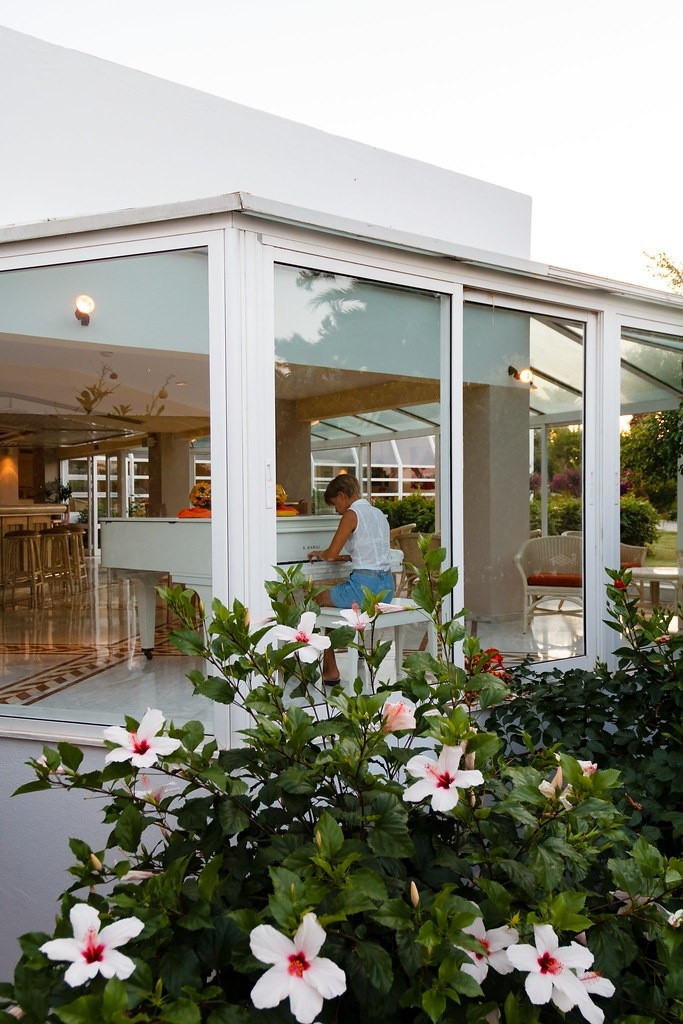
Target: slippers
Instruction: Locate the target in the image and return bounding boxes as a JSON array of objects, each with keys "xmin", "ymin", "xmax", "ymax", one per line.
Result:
[{"xmin": 322, "ymin": 679, "xmax": 340, "ymax": 687}]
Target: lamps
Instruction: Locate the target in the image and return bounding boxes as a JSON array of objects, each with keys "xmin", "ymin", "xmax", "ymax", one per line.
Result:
[
  {"xmin": 275, "ymin": 363, "xmax": 291, "ymax": 378},
  {"xmin": 508, "ymin": 366, "xmax": 532, "ymax": 383},
  {"xmin": 75, "ymin": 294, "xmax": 94, "ymax": 327}
]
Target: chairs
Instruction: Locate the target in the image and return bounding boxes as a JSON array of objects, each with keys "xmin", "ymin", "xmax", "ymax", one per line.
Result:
[
  {"xmin": 620, "ymin": 543, "xmax": 648, "ymax": 602},
  {"xmin": 392, "ymin": 533, "xmax": 441, "ymax": 600},
  {"xmin": 513, "ymin": 535, "xmax": 582, "ymax": 634},
  {"xmin": 558, "ymin": 531, "xmax": 582, "ymax": 612},
  {"xmin": 390, "ymin": 522, "xmax": 417, "ymax": 591}
]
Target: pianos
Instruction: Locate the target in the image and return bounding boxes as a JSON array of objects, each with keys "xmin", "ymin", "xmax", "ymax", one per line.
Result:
[{"xmin": 100, "ymin": 516, "xmax": 405, "ymax": 660}]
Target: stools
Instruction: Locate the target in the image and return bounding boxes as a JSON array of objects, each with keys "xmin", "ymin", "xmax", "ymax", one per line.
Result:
[
  {"xmin": 39, "ymin": 528, "xmax": 74, "ymax": 597},
  {"xmin": 52, "ymin": 525, "xmax": 88, "ymax": 592},
  {"xmin": 314, "ymin": 598, "xmax": 437, "ymax": 694},
  {"xmin": 2, "ymin": 529, "xmax": 45, "ymax": 613}
]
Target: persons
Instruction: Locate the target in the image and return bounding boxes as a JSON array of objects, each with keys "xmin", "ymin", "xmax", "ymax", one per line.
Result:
[{"xmin": 308, "ymin": 474, "xmax": 395, "ymax": 686}]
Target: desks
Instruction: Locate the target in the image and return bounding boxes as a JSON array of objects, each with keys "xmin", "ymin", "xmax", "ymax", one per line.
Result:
[{"xmin": 629, "ymin": 566, "xmax": 679, "ymax": 610}]
